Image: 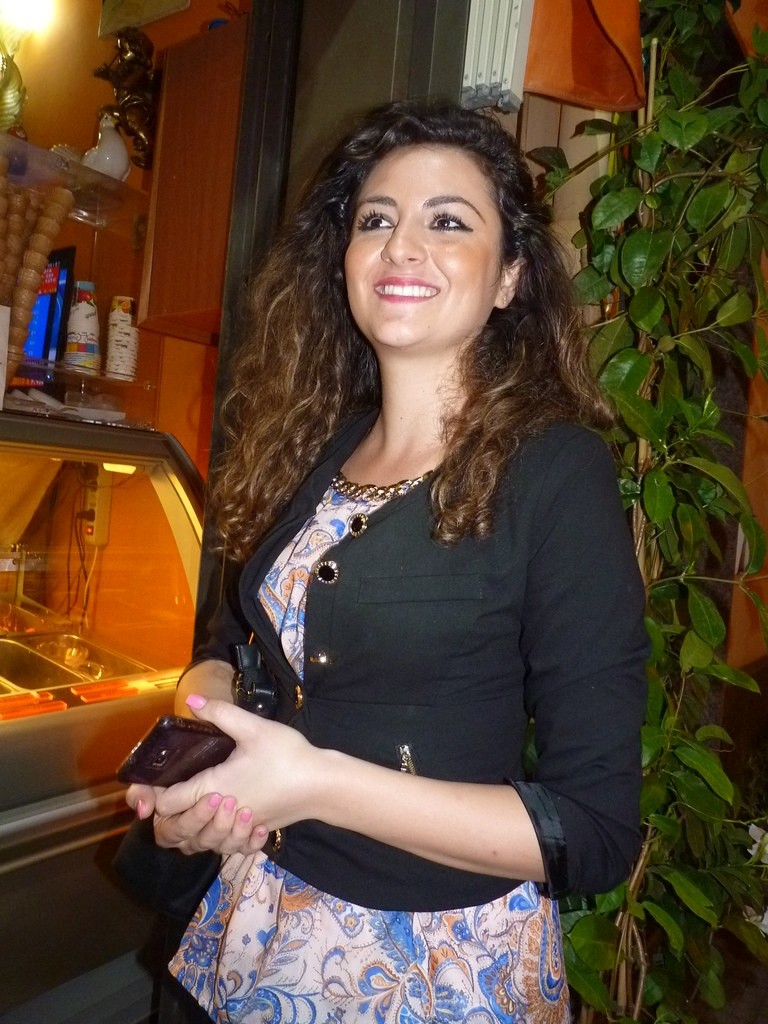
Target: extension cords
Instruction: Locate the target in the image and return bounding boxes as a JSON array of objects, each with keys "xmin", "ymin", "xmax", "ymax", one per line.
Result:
[{"xmin": 82, "ymin": 461, "xmax": 112, "ymax": 546}]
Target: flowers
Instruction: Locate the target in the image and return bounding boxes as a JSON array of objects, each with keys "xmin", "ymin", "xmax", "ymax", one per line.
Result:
[{"xmin": 524, "ymin": 0, "xmax": 768, "ymax": 1024}]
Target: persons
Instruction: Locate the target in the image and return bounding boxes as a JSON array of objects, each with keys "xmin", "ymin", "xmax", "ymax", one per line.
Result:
[{"xmin": 117, "ymin": 91, "xmax": 660, "ymax": 1024}]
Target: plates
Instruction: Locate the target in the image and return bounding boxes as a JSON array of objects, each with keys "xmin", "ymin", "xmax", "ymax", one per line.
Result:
[{"xmin": 60, "ymin": 407, "xmax": 125, "ymax": 421}]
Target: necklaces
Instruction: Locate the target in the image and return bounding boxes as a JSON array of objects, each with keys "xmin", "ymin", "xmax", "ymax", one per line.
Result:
[{"xmin": 325, "ymin": 467, "xmax": 434, "ymax": 507}]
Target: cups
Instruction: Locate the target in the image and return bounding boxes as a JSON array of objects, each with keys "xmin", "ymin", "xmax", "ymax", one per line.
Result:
[
  {"xmin": 106, "ymin": 296, "xmax": 140, "ymax": 382},
  {"xmin": 95, "ymin": 395, "xmax": 122, "ymax": 412},
  {"xmin": 64, "ymin": 280, "xmax": 101, "ymax": 376},
  {"xmin": 65, "ymin": 392, "xmax": 94, "ymax": 409}
]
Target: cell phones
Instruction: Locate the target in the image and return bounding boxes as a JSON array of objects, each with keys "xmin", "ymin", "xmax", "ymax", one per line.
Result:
[{"xmin": 116, "ymin": 715, "xmax": 236, "ymax": 789}]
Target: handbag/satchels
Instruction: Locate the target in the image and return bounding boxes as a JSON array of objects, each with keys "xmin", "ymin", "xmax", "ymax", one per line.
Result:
[{"xmin": 109, "ymin": 632, "xmax": 280, "ymax": 920}]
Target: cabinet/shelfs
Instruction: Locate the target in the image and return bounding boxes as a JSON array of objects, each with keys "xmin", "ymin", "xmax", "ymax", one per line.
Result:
[{"xmin": 0, "ymin": 132, "xmax": 164, "ymax": 432}]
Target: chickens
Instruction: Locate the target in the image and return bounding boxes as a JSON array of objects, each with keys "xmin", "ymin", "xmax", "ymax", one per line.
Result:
[{"xmin": 49, "ymin": 113, "xmax": 132, "ymax": 183}]
